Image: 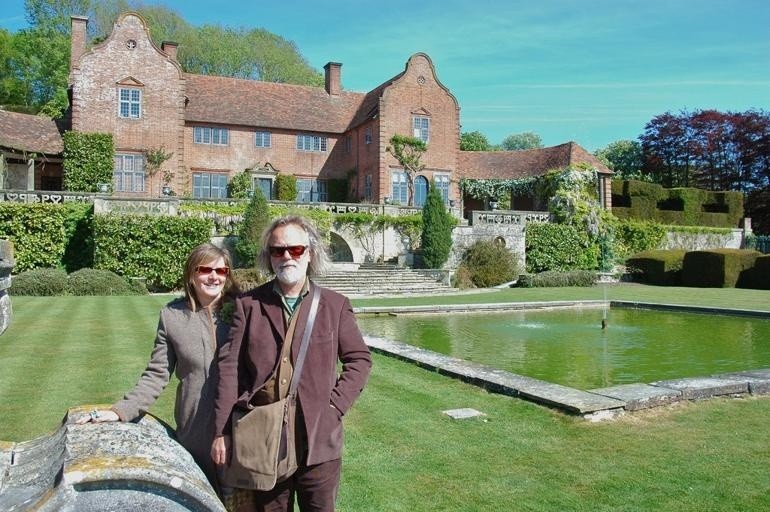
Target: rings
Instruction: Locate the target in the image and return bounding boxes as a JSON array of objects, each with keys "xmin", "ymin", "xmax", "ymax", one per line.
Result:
[{"xmin": 90, "ymin": 411, "xmax": 98, "ymax": 419}]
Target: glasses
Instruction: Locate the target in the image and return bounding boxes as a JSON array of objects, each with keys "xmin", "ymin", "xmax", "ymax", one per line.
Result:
[
  {"xmin": 194, "ymin": 265, "xmax": 229, "ymax": 275},
  {"xmin": 268, "ymin": 245, "xmax": 309, "ymax": 259}
]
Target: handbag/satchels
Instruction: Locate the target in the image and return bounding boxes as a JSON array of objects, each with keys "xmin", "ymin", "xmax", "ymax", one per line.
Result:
[{"xmin": 216, "ymin": 397, "xmax": 298, "ymax": 492}]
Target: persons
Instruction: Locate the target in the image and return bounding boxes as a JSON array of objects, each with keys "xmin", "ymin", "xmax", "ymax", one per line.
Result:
[
  {"xmin": 212, "ymin": 214, "xmax": 373, "ymax": 512},
  {"xmin": 76, "ymin": 242, "xmax": 256, "ymax": 512}
]
